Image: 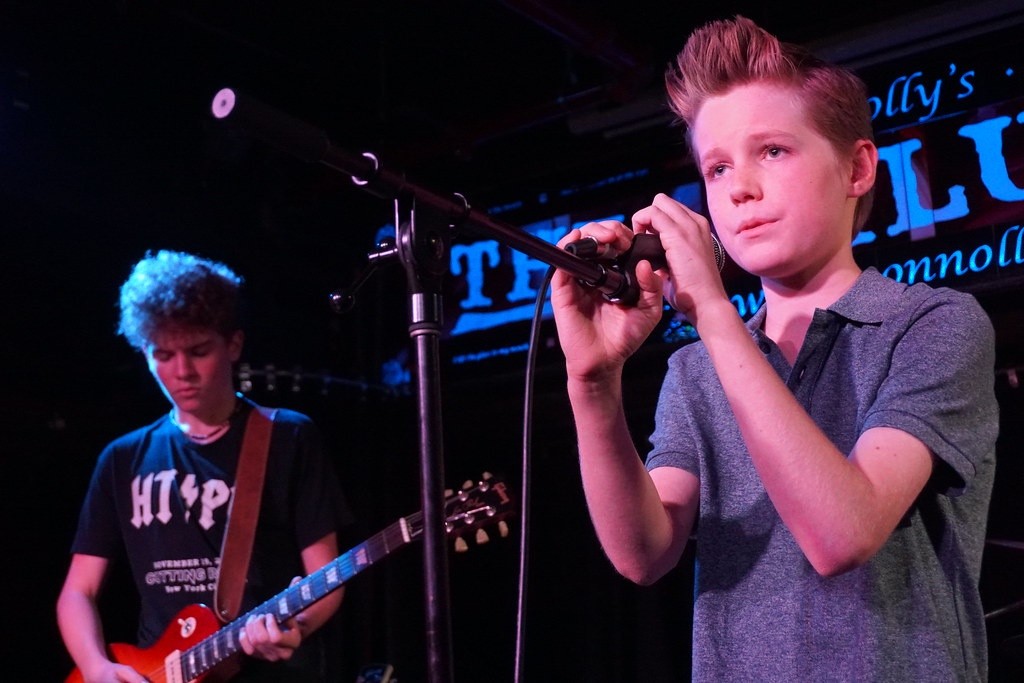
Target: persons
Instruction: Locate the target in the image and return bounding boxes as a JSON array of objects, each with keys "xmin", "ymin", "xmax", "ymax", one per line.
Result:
[
  {"xmin": 56, "ymin": 245, "xmax": 344, "ymax": 683},
  {"xmin": 551, "ymin": 13, "xmax": 998, "ymax": 683}
]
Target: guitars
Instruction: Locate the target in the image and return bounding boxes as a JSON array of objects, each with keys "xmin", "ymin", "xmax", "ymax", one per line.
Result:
[{"xmin": 59, "ymin": 469, "xmax": 515, "ymax": 683}]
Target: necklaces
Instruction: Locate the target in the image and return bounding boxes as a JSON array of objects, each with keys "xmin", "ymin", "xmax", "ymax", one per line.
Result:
[{"xmin": 185, "ymin": 419, "xmax": 228, "ymax": 439}]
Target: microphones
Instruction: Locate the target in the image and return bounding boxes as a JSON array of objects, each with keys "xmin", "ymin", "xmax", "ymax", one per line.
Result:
[{"xmin": 565, "ymin": 231, "xmax": 727, "ymax": 274}]
